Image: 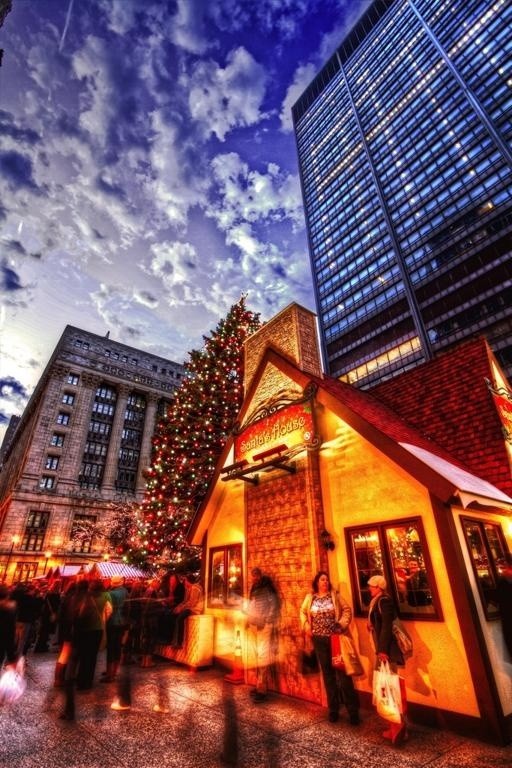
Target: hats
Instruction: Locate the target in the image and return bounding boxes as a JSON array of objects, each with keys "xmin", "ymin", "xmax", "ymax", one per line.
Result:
[
  {"xmin": 367, "ymin": 575, "xmax": 387, "ymax": 591},
  {"xmin": 111, "ymin": 576, "xmax": 123, "ymax": 586}
]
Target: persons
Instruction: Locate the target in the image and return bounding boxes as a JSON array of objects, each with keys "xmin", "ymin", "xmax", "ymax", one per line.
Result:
[
  {"xmin": 299, "ymin": 571, "xmax": 364, "ymax": 725},
  {"xmin": 366, "ymin": 574, "xmax": 414, "ymax": 748},
  {"xmin": 406, "ymin": 558, "xmax": 430, "ymax": 608},
  {"xmin": 480, "ymin": 552, "xmax": 511, "ymax": 666},
  {"xmin": 394, "ymin": 558, "xmax": 416, "ymax": 598},
  {"xmin": 1, "ymin": 563, "xmax": 284, "ymax": 767}
]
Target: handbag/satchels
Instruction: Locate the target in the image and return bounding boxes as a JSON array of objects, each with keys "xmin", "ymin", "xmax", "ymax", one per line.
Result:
[
  {"xmin": 49, "ymin": 612, "xmax": 57, "ymax": 623},
  {"xmin": 391, "ymin": 617, "xmax": 412, "ymax": 656},
  {"xmin": 98, "ymin": 629, "xmax": 107, "ymax": 652},
  {"xmin": 343, "ymin": 652, "xmax": 363, "ymax": 676},
  {"xmin": 372, "ymin": 670, "xmax": 408, "ymax": 715},
  {"xmin": 301, "ymin": 650, "xmax": 320, "ymax": 674}
]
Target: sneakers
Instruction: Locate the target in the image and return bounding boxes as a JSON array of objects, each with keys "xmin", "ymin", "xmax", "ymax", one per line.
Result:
[
  {"xmin": 383, "ymin": 723, "xmax": 408, "ymax": 744},
  {"xmin": 100, "ymin": 676, "xmax": 117, "ymax": 682},
  {"xmin": 249, "ymin": 687, "xmax": 270, "ymax": 703},
  {"xmin": 328, "ymin": 711, "xmax": 338, "ymax": 723},
  {"xmin": 350, "ymin": 711, "xmax": 359, "ymax": 726}
]
